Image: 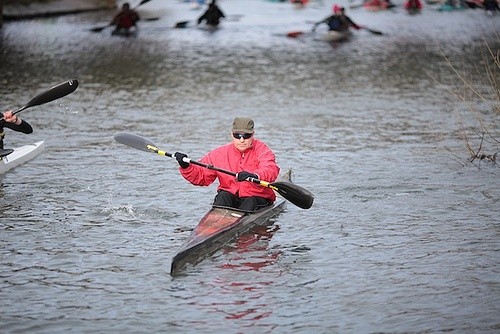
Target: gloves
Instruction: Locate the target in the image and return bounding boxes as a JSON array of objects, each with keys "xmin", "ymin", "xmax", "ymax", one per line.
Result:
[
  {"xmin": 174, "ymin": 152, "xmax": 189, "ymax": 169},
  {"xmin": 235, "ymin": 171, "xmax": 258, "ymax": 182}
]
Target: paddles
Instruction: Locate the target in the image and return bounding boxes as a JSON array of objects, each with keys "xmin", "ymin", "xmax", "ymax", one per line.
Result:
[
  {"xmin": 0, "ymin": 78, "xmax": 78, "ymax": 122},
  {"xmin": 114, "ymin": 133, "xmax": 314, "ymax": 209}
]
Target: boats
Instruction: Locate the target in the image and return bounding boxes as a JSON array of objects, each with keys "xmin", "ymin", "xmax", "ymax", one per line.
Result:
[
  {"xmin": 0, "ymin": 140, "xmax": 46, "ymax": 174},
  {"xmin": 112, "ymin": 22, "xmax": 139, "ymax": 38},
  {"xmin": 171, "ymin": 168, "xmax": 294, "ymax": 263},
  {"xmin": 321, "ymin": 29, "xmax": 353, "ymax": 43}
]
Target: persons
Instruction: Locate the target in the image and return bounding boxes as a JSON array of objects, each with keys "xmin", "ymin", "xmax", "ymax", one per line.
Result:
[
  {"xmin": 324, "ymin": 0, "xmax": 499, "ymax": 31},
  {"xmin": 110, "ymin": 1, "xmax": 224, "ymax": 31},
  {"xmin": 0, "ymin": 110, "xmax": 33, "ymax": 148},
  {"xmin": 173, "ymin": 116, "xmax": 280, "ymax": 211}
]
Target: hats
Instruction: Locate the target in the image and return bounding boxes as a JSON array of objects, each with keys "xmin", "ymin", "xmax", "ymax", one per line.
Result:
[{"xmin": 231, "ymin": 118, "xmax": 254, "ymax": 134}]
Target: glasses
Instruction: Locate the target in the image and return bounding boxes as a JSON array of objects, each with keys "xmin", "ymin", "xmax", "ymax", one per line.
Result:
[{"xmin": 232, "ymin": 133, "xmax": 252, "ymax": 139}]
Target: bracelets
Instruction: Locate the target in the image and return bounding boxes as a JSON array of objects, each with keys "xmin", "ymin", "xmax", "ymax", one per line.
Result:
[{"xmin": 12, "ymin": 116, "xmax": 18, "ymax": 124}]
